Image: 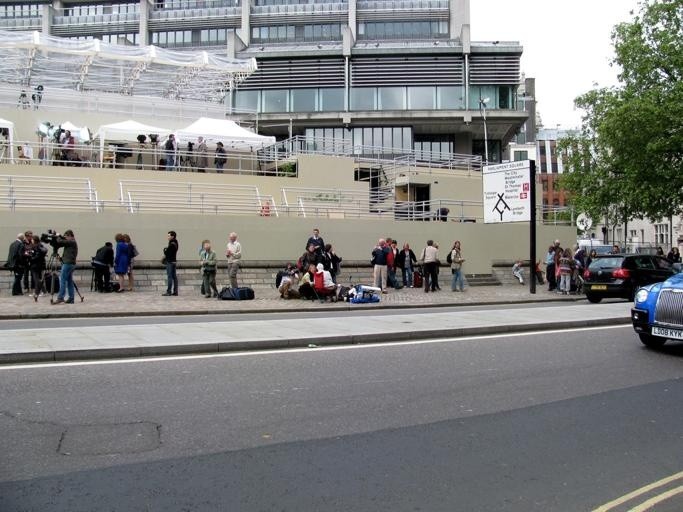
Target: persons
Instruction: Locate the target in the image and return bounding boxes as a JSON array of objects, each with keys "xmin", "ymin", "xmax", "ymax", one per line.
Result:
[
  {"xmin": 16, "ymin": 129, "xmax": 228, "ymax": 174},
  {"xmin": 370, "ymin": 237, "xmax": 468, "ymax": 292},
  {"xmin": 432, "ymin": 207, "xmax": 450, "ymax": 222},
  {"xmin": 545, "ymin": 239, "xmax": 619, "ymax": 296},
  {"xmin": 16, "ymin": 89, "xmax": 30, "ymax": 108},
  {"xmin": 161, "ymin": 231, "xmax": 178, "ymax": 296},
  {"xmin": 225, "ymin": 231, "xmax": 241, "ymax": 289},
  {"xmin": 3, "ymin": 229, "xmax": 139, "ymax": 306},
  {"xmin": 667, "ymin": 247, "xmax": 673, "ymax": 262},
  {"xmin": 199, "ymin": 240, "xmax": 213, "ymax": 295},
  {"xmin": 535, "ymin": 259, "xmax": 545, "ymax": 285},
  {"xmin": 198, "ymin": 242, "xmax": 219, "ymax": 298},
  {"xmin": 259, "ymin": 200, "xmax": 270, "ymax": 216},
  {"xmin": 670, "ymin": 247, "xmax": 680, "ymax": 263},
  {"xmin": 510, "ymin": 259, "xmax": 526, "ymax": 285},
  {"xmin": 655, "ymin": 246, "xmax": 663, "ymax": 256},
  {"xmin": 31, "ymin": 84, "xmax": 43, "ymax": 110},
  {"xmin": 275, "ymin": 228, "xmax": 343, "ymax": 304}
]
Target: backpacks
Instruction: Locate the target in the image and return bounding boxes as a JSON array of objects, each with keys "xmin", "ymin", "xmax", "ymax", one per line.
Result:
[
  {"xmin": 447, "ymin": 250, "xmax": 452, "ymax": 264},
  {"xmin": 220, "ymin": 284, "xmax": 234, "ymax": 299}
]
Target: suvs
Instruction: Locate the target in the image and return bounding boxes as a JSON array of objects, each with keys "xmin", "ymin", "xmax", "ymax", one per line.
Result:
[
  {"xmin": 631, "ymin": 271, "xmax": 683, "ymax": 351},
  {"xmin": 582, "ymin": 254, "xmax": 681, "ymax": 302}
]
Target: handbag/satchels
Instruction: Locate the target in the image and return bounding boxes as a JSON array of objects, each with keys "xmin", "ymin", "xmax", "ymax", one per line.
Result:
[{"xmin": 235, "ymin": 287, "xmax": 255, "ymax": 299}]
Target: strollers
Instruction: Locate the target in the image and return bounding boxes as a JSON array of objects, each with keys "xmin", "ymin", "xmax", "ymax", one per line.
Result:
[{"xmin": 571, "ymin": 265, "xmax": 585, "ymax": 295}]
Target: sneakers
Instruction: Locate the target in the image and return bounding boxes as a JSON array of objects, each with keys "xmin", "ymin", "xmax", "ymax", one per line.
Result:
[
  {"xmin": 205, "ymin": 292, "xmax": 218, "ymax": 297},
  {"xmin": 162, "ymin": 293, "xmax": 171, "ymax": 296},
  {"xmin": 403, "ymin": 285, "xmax": 413, "ymax": 287},
  {"xmin": 452, "ymin": 288, "xmax": 467, "ymax": 292},
  {"xmin": 53, "ymin": 298, "xmax": 74, "ymax": 305},
  {"xmin": 97, "ymin": 289, "xmax": 133, "ymax": 293},
  {"xmin": 424, "ymin": 287, "xmax": 441, "ymax": 292},
  {"xmin": 172, "ymin": 293, "xmax": 178, "ymax": 296}
]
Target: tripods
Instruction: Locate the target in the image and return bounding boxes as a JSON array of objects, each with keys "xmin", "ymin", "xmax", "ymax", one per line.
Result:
[
  {"xmin": 180, "ymin": 153, "xmax": 194, "ymax": 172},
  {"xmin": 35, "ymin": 254, "xmax": 84, "ymax": 303},
  {"xmin": 53, "ymin": 140, "xmax": 68, "ymax": 166}
]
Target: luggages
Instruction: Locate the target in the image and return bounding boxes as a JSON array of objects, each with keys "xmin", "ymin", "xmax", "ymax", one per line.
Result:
[{"xmin": 414, "ymin": 270, "xmax": 423, "ymax": 288}]
